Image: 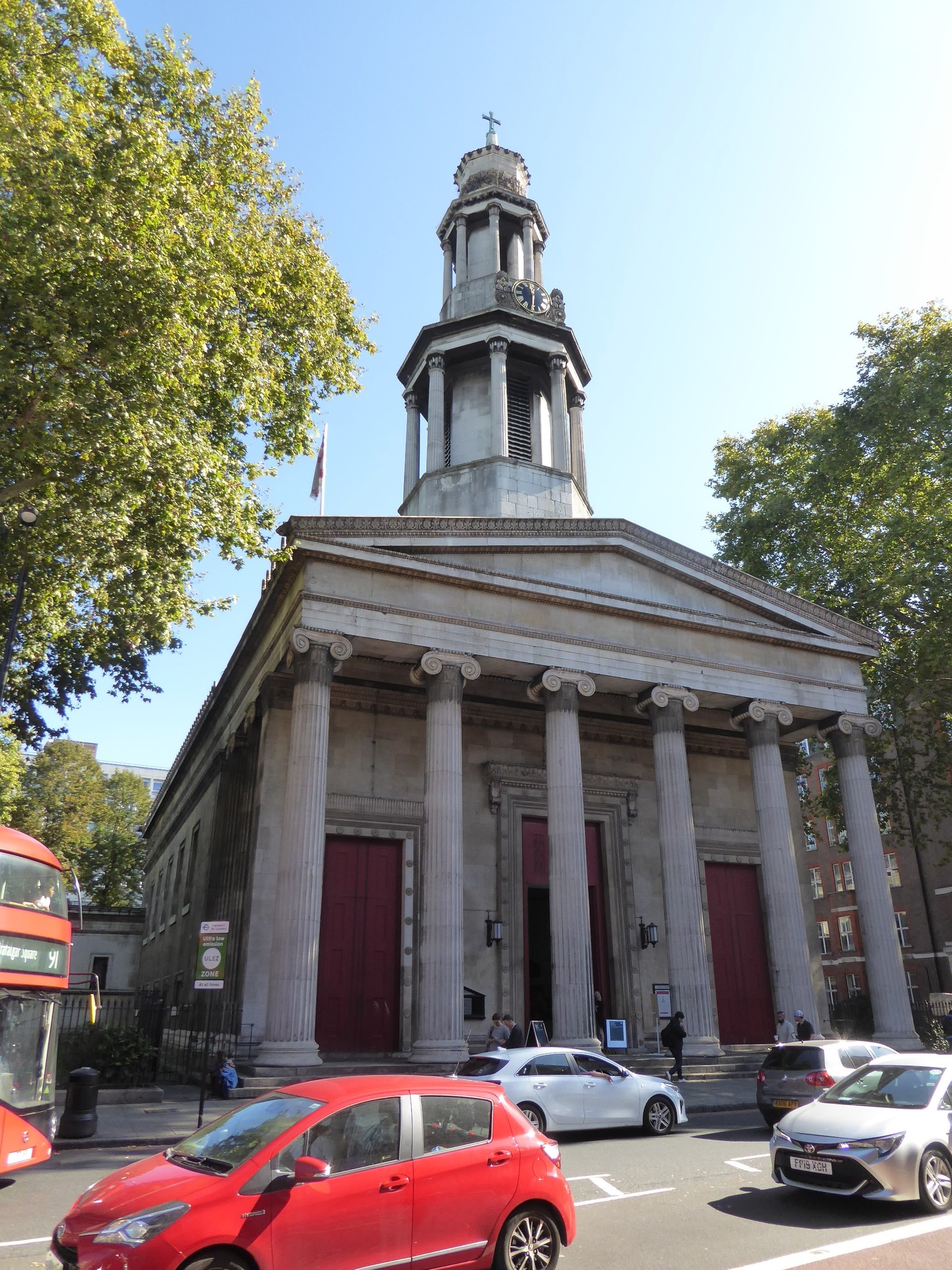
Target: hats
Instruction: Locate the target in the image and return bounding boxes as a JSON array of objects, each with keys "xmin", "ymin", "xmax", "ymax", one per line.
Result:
[{"xmin": 794, "ymin": 1008, "xmax": 803, "ymax": 1017}]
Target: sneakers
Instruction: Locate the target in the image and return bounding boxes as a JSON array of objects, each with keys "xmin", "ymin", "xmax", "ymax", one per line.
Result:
[
  {"xmin": 665, "ymin": 1071, "xmax": 671, "ymax": 1081},
  {"xmin": 678, "ymin": 1078, "xmax": 688, "ymax": 1083}
]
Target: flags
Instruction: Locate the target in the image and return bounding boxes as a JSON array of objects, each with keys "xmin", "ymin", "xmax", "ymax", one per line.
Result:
[{"xmin": 309, "ymin": 431, "xmax": 326, "ymax": 500}]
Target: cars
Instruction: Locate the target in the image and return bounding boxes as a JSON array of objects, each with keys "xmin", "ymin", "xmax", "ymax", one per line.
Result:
[
  {"xmin": 769, "ymin": 1054, "xmax": 952, "ymax": 1213},
  {"xmin": 453, "ymin": 1047, "xmax": 689, "ymax": 1138},
  {"xmin": 46, "ymin": 1074, "xmax": 575, "ymax": 1270}
]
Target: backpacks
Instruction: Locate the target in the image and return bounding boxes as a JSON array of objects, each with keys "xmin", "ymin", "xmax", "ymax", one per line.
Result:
[{"xmin": 660, "ymin": 1023, "xmax": 680, "ymax": 1047}]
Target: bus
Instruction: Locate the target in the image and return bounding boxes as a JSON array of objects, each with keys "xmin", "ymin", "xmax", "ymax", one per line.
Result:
[{"xmin": 0, "ymin": 825, "xmax": 101, "ymax": 1173}]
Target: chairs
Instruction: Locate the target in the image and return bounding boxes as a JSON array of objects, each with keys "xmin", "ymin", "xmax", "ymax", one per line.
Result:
[
  {"xmin": 362, "ymin": 1103, "xmax": 404, "ymax": 1164},
  {"xmin": 893, "ymin": 1070, "xmax": 928, "ymax": 1105},
  {"xmin": 310, "ymin": 1111, "xmax": 353, "ymax": 1168}
]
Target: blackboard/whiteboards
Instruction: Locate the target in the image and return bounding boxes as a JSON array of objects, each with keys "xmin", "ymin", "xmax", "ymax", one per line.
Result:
[{"xmin": 525, "ymin": 1021, "xmax": 549, "ymax": 1047}]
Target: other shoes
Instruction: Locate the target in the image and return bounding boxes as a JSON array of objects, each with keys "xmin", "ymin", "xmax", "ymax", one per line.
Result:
[{"xmin": 207, "ymin": 1093, "xmax": 223, "ymax": 1100}]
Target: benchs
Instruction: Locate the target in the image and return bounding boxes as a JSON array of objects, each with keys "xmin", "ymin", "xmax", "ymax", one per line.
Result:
[{"xmin": 424, "ymin": 1125, "xmax": 486, "ymax": 1154}]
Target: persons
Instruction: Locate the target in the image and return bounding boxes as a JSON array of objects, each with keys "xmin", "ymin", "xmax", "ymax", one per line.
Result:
[
  {"xmin": 500, "ymin": 1015, "xmax": 525, "ymax": 1049},
  {"xmin": 775, "ymin": 1012, "xmax": 794, "ymax": 1043},
  {"xmin": 208, "ymin": 1050, "xmax": 238, "ymax": 1100},
  {"xmin": 665, "ymin": 1011, "xmax": 687, "ymax": 1082},
  {"xmin": 595, "ymin": 991, "xmax": 605, "ymax": 1048},
  {"xmin": 568, "ymin": 1057, "xmax": 614, "ymax": 1083},
  {"xmin": 485, "ymin": 1013, "xmax": 510, "ymax": 1052},
  {"xmin": 943, "ymin": 1010, "xmax": 952, "ymax": 1034},
  {"xmin": 791, "ymin": 1009, "xmax": 814, "ymax": 1041}
]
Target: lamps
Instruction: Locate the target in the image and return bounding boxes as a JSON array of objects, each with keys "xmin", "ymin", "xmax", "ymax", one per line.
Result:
[
  {"xmin": 635, "ymin": 915, "xmax": 659, "ymax": 950},
  {"xmin": 484, "ymin": 909, "xmax": 504, "ymax": 948}
]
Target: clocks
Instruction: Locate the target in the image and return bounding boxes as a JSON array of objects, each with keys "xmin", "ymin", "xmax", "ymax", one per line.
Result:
[{"xmin": 510, "ymin": 279, "xmax": 552, "ymax": 314}]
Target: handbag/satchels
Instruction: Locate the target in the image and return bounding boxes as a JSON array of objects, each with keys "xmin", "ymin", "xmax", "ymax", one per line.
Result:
[{"xmin": 483, "ymin": 1043, "xmax": 489, "ymax": 1053}]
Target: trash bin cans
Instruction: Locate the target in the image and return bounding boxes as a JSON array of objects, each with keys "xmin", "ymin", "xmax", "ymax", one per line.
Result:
[{"xmin": 58, "ymin": 1067, "xmax": 100, "ymax": 1138}]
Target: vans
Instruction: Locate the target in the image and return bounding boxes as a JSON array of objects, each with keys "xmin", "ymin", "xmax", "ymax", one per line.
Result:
[{"xmin": 756, "ymin": 1040, "xmax": 902, "ymax": 1127}]
